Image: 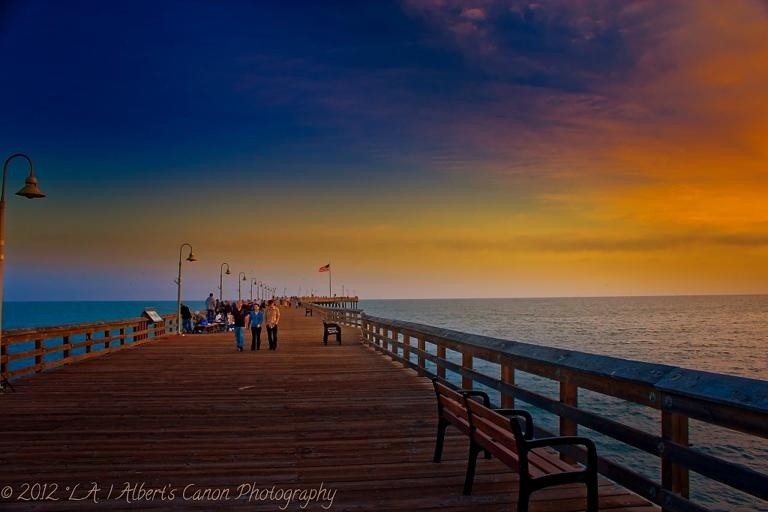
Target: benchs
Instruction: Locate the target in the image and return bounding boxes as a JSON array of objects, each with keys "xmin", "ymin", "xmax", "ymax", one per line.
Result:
[
  {"xmin": 428, "ymin": 378, "xmax": 600, "ymax": 512},
  {"xmin": 322, "ymin": 318, "xmax": 341, "ymax": 344}
]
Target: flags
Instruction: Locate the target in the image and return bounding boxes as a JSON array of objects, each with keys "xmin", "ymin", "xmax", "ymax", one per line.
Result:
[{"xmin": 319, "ymin": 265, "xmax": 329, "ymax": 272}]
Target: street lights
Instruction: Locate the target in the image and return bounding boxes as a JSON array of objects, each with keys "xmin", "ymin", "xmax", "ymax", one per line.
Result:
[
  {"xmin": 174, "ymin": 242, "xmax": 274, "ymax": 335},
  {"xmin": 0, "ymin": 154, "xmax": 48, "ymax": 321}
]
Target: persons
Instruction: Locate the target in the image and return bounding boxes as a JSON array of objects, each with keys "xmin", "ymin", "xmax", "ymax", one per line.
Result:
[
  {"xmin": 230, "ymin": 300, "xmax": 249, "ymax": 352},
  {"xmin": 181, "ymin": 293, "xmax": 302, "ymax": 334},
  {"xmin": 249, "ymin": 304, "xmax": 263, "ymax": 350},
  {"xmin": 263, "ymin": 300, "xmax": 280, "ymax": 350}
]
